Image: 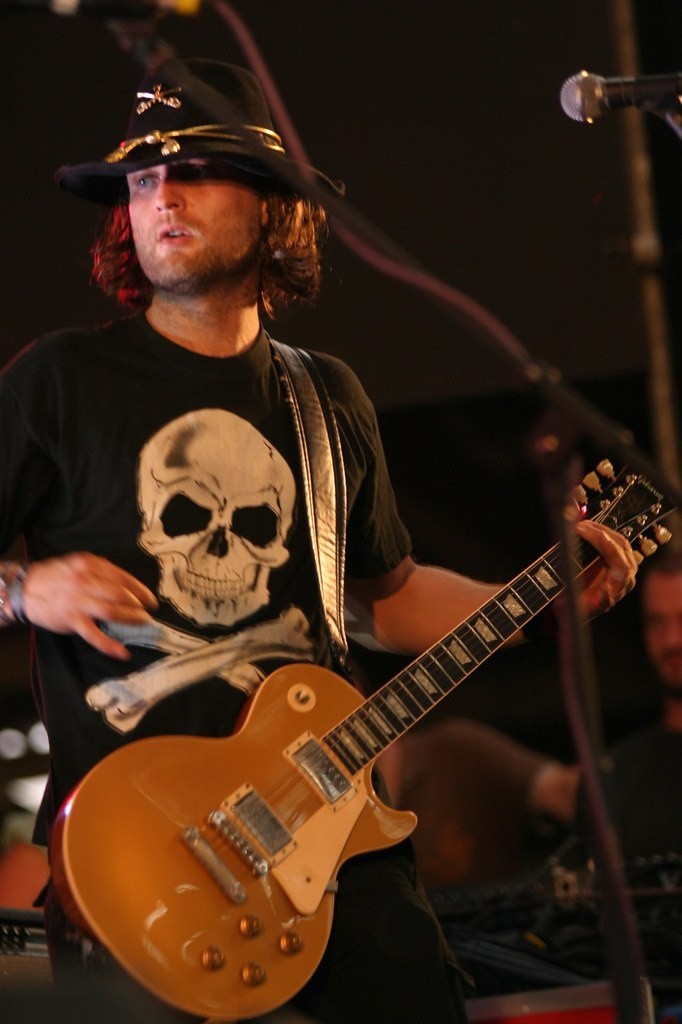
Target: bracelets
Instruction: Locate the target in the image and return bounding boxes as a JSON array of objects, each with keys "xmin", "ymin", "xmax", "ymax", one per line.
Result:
[{"xmin": 0, "ymin": 562, "xmax": 28, "ymax": 627}]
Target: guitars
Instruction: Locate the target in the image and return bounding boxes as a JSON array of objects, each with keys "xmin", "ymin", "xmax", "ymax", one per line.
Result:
[{"xmin": 42, "ymin": 448, "xmax": 682, "ymax": 1024}]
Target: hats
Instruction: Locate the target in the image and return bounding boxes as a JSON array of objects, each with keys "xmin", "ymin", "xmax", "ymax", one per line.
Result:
[{"xmin": 53, "ymin": 58, "xmax": 345, "ymax": 204}]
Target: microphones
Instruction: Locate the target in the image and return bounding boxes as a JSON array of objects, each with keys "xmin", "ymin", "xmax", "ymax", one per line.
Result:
[{"xmin": 560, "ymin": 69, "xmax": 682, "ymax": 124}]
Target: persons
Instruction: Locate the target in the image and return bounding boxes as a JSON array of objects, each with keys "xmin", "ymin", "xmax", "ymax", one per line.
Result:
[
  {"xmin": 0, "ymin": 56, "xmax": 645, "ymax": 1024},
  {"xmin": 362, "ymin": 556, "xmax": 682, "ymax": 1024}
]
